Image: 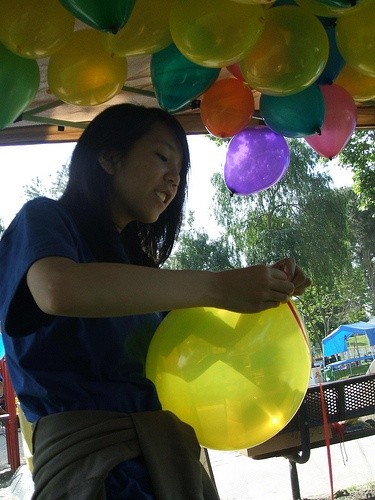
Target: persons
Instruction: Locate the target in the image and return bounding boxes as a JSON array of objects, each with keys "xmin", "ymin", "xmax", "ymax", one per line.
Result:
[{"xmin": 0, "ymin": 104, "xmax": 312, "ymax": 500}]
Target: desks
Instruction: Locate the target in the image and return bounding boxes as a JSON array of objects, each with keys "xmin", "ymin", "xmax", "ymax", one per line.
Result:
[{"xmin": 326, "ymin": 356, "xmax": 375, "ymax": 381}]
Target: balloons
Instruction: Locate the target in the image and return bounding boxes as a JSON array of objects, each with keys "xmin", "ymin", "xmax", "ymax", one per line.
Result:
[
  {"xmin": 224, "ymin": 125, "xmax": 290, "ymax": 197},
  {"xmin": 0, "ymin": 0, "xmax": 375, "ymax": 162},
  {"xmin": 146, "ymin": 295, "xmax": 312, "ymax": 451}
]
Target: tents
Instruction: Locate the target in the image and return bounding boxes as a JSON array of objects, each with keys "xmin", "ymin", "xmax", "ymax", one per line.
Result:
[{"xmin": 321, "ymin": 321, "xmax": 375, "ymax": 369}]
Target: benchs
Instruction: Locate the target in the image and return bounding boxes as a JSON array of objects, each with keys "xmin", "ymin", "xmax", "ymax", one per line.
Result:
[{"xmin": 235, "ymin": 374, "xmax": 375, "ymax": 500}]
